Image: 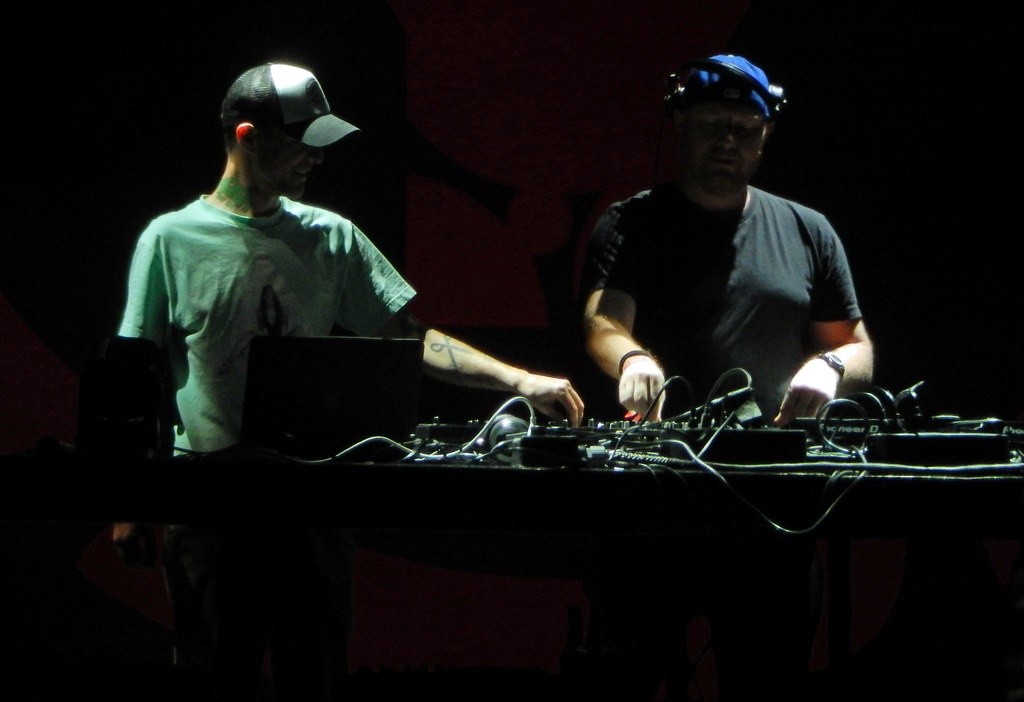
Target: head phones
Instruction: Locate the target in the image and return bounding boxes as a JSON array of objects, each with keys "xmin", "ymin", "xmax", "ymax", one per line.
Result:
[{"xmin": 483, "ymin": 397, "xmax": 538, "ymax": 465}]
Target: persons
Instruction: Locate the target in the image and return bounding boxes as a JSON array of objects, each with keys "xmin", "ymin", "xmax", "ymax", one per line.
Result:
[
  {"xmin": 584, "ymin": 51, "xmax": 876, "ymax": 702},
  {"xmin": 116, "ymin": 59, "xmax": 585, "ymax": 702}
]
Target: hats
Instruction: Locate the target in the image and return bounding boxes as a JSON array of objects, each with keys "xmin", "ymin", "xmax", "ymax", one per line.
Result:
[
  {"xmin": 221, "ymin": 60, "xmax": 361, "ymax": 147},
  {"xmin": 679, "ymin": 55, "xmax": 772, "ymax": 119}
]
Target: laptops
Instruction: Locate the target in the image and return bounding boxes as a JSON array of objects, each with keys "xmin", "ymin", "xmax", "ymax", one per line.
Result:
[{"xmin": 238, "ymin": 336, "xmax": 425, "ymax": 463}]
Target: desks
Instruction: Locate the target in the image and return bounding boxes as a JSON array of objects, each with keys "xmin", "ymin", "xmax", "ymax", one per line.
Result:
[{"xmin": 183, "ymin": 457, "xmax": 1024, "ymax": 702}]
